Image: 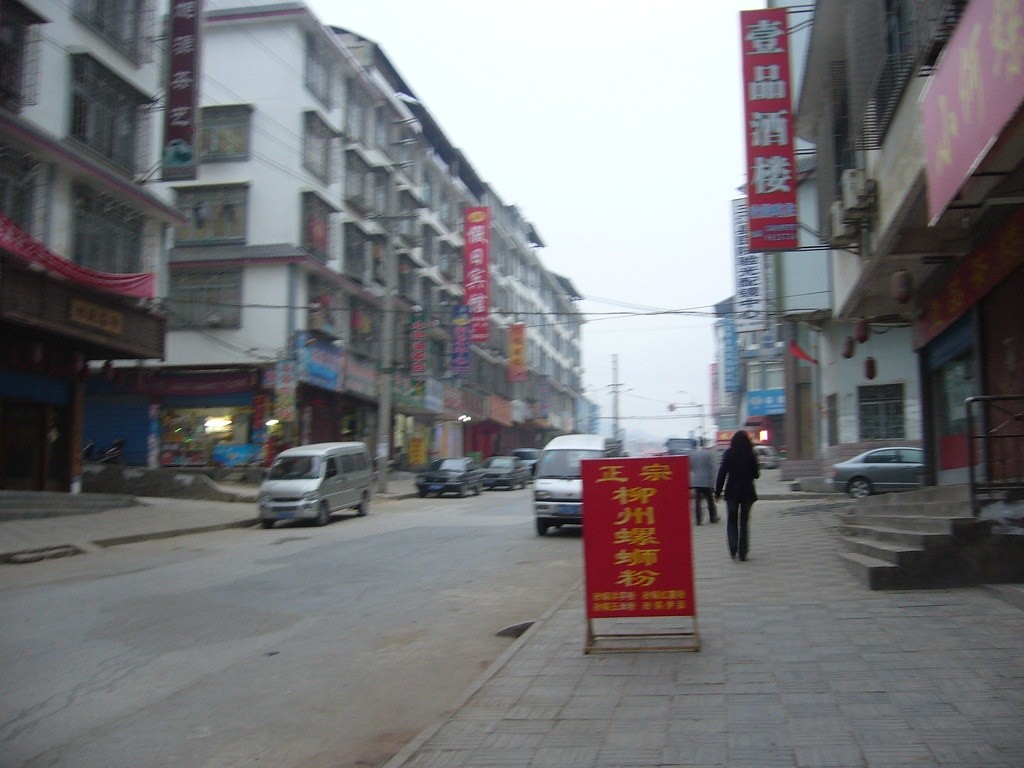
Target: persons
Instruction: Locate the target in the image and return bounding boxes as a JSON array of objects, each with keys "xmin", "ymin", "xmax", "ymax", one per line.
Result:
[
  {"xmin": 715, "ymin": 430, "xmax": 761, "ymax": 560},
  {"xmin": 690, "ymin": 439, "xmax": 721, "ymax": 525}
]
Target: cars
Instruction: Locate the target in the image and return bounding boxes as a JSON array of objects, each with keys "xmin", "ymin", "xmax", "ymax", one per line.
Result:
[
  {"xmin": 415, "ymin": 457, "xmax": 487, "ymax": 498},
  {"xmin": 479, "ymin": 455, "xmax": 531, "ymax": 490},
  {"xmin": 753, "ymin": 445, "xmax": 781, "ymax": 469},
  {"xmin": 827, "ymin": 445, "xmax": 925, "ymax": 500}
]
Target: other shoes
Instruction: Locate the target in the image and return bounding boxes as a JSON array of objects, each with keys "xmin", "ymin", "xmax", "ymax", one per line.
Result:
[
  {"xmin": 697, "ymin": 521, "xmax": 702, "ymax": 525},
  {"xmin": 714, "ymin": 514, "xmax": 721, "ymax": 522}
]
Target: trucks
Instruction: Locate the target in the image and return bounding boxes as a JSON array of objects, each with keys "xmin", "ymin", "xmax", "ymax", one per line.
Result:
[{"xmin": 664, "ymin": 438, "xmax": 698, "ymax": 454}]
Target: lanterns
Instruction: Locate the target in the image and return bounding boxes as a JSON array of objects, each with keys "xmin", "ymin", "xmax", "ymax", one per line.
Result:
[
  {"xmin": 843, "ymin": 336, "xmax": 855, "ymax": 358},
  {"xmin": 855, "ymin": 316, "xmax": 870, "ymax": 343},
  {"xmin": 865, "ymin": 356, "xmax": 876, "ymax": 380},
  {"xmin": 893, "ymin": 268, "xmax": 914, "ymax": 305}
]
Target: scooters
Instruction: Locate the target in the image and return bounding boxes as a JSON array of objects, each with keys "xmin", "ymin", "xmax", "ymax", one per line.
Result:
[{"xmin": 80, "ymin": 438, "xmax": 130, "ymax": 466}]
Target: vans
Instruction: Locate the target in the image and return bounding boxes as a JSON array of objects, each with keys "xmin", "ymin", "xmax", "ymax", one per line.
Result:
[
  {"xmin": 529, "ymin": 432, "xmax": 630, "ymax": 536},
  {"xmin": 258, "ymin": 442, "xmax": 375, "ymax": 529},
  {"xmin": 510, "ymin": 447, "xmax": 541, "ymax": 470}
]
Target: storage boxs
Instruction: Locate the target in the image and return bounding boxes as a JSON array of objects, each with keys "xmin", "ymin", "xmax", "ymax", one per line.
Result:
[
  {"xmin": 826, "ymin": 201, "xmax": 856, "ymax": 249},
  {"xmin": 837, "ymin": 168, "xmax": 868, "ymax": 220}
]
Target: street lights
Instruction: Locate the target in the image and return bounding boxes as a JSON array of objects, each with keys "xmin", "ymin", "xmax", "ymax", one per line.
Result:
[{"xmin": 669, "ymin": 402, "xmax": 706, "ymax": 447}]
